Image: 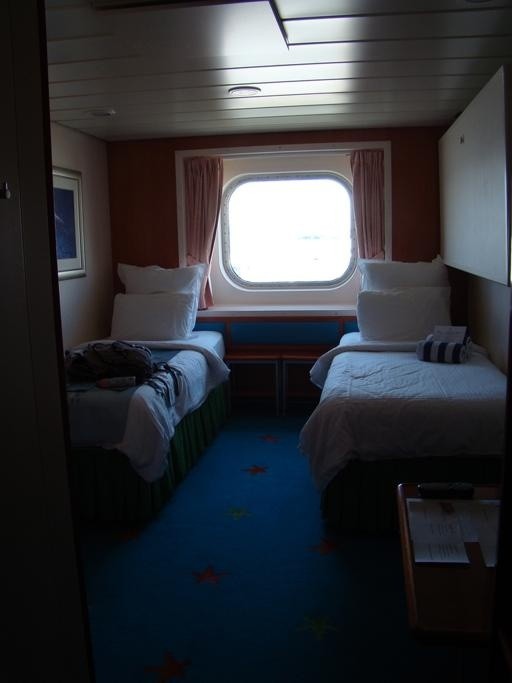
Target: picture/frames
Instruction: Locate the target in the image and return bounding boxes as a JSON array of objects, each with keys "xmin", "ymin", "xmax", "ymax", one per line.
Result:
[{"xmin": 52, "ymin": 165, "xmax": 87, "ymax": 281}]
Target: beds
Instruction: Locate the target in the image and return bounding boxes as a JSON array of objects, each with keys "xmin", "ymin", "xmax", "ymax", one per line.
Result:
[
  {"xmin": 65, "ymin": 331, "xmax": 230, "ymax": 530},
  {"xmin": 298, "ymin": 332, "xmax": 507, "ymax": 534}
]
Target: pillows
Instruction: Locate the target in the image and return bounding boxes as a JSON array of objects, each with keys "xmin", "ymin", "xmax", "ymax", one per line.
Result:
[
  {"xmin": 354, "ymin": 254, "xmax": 451, "ymax": 341},
  {"xmin": 110, "ymin": 262, "xmax": 213, "ymax": 341}
]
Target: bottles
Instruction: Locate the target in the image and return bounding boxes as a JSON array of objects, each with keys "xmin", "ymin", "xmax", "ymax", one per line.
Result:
[{"xmin": 99, "ymin": 376, "xmax": 136, "ymax": 387}]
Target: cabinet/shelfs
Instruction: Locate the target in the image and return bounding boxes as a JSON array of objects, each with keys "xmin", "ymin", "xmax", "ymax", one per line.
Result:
[{"xmin": 223, "ymin": 352, "xmax": 329, "ymax": 417}]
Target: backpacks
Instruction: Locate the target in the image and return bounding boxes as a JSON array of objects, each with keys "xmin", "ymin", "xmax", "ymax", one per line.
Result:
[{"xmin": 64, "ymin": 340, "xmax": 154, "ymax": 384}]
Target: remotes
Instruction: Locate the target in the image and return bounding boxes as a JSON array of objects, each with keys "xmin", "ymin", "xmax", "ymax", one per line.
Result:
[{"xmin": 417, "ymin": 480, "xmax": 475, "ymax": 498}]
